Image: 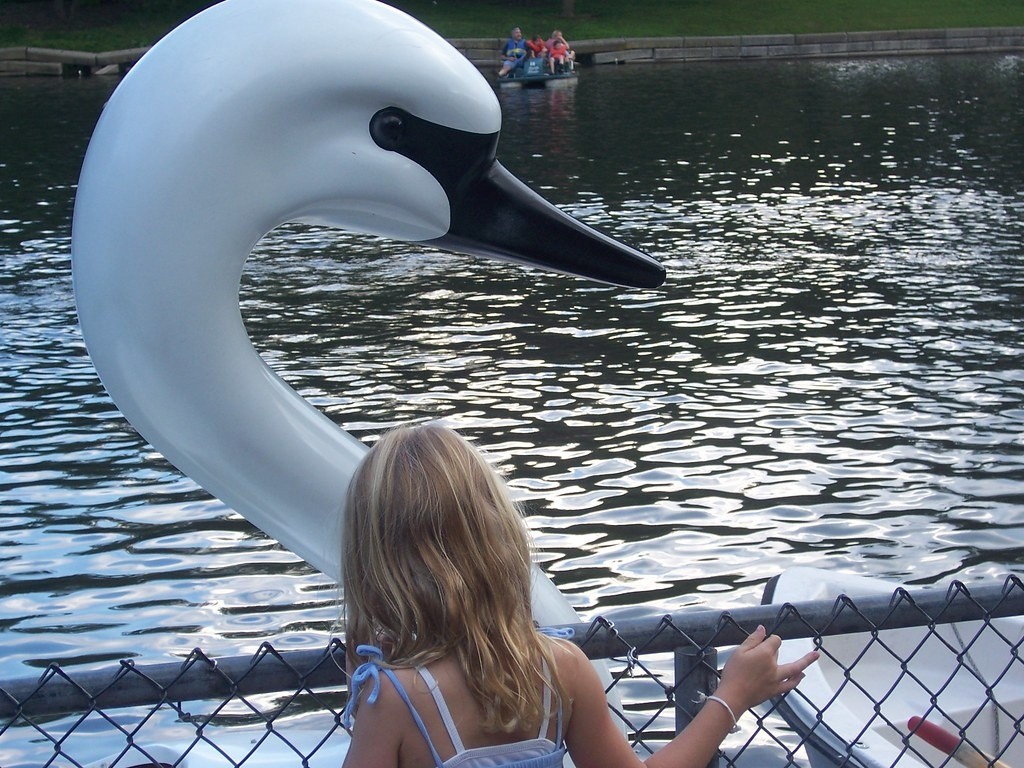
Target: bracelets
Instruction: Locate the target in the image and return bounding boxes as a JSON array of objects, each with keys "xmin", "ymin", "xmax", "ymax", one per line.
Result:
[{"xmin": 691, "ymin": 691, "xmax": 741, "ymax": 734}]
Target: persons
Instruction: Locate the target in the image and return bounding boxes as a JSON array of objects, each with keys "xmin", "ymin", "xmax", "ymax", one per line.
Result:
[
  {"xmin": 342, "ymin": 426, "xmax": 820, "ymax": 768},
  {"xmin": 496, "ymin": 26, "xmax": 532, "ymax": 79},
  {"xmin": 526, "ymin": 31, "xmax": 576, "ymax": 75}
]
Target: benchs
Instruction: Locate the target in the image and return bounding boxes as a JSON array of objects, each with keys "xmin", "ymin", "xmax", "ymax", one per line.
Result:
[{"xmin": 508, "ymin": 62, "xmax": 582, "ymax": 77}]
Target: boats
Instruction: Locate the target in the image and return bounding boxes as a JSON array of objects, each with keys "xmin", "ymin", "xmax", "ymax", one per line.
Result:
[
  {"xmin": 494, "ymin": 56, "xmax": 582, "ymax": 91},
  {"xmin": 755, "ymin": 565, "xmax": 1024, "ymax": 768}
]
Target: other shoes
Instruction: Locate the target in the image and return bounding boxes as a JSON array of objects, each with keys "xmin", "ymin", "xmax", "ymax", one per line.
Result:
[
  {"xmin": 556, "ymin": 64, "xmax": 564, "ymax": 71},
  {"xmin": 493, "ymin": 69, "xmax": 502, "ymax": 78},
  {"xmin": 548, "ymin": 73, "xmax": 555, "ymax": 76}
]
